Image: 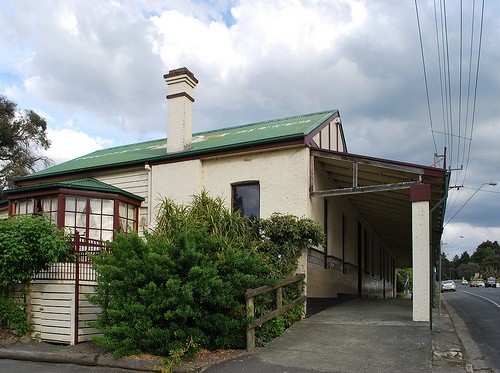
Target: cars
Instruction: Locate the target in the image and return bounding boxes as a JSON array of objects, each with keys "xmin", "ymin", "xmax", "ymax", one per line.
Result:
[
  {"xmin": 470, "ymin": 280, "xmax": 478, "ymax": 287},
  {"xmin": 461, "ymin": 280, "xmax": 468, "ymax": 285},
  {"xmin": 476, "ymin": 281, "xmax": 485, "ymax": 287},
  {"xmin": 441, "ymin": 279, "xmax": 456, "ymax": 292}
]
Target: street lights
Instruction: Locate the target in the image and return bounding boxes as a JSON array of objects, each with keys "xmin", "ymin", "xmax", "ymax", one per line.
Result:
[{"xmin": 438, "ymin": 182, "xmax": 498, "ymax": 316}]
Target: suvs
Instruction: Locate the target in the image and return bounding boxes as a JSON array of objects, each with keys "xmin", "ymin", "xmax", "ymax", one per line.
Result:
[{"xmin": 485, "ymin": 277, "xmax": 496, "ymax": 288}]
